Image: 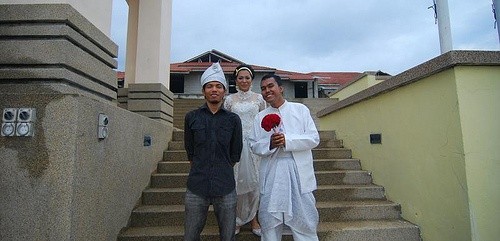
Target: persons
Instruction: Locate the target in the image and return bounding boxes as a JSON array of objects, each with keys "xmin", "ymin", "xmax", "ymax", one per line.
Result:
[
  {"xmin": 249, "ymin": 74, "xmax": 322, "ymax": 241},
  {"xmin": 223, "ymin": 64, "xmax": 265, "ymax": 236},
  {"xmin": 183, "ymin": 62, "xmax": 245, "ymax": 241}
]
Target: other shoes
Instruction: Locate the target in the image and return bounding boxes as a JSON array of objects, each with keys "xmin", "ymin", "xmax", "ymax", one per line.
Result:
[
  {"xmin": 251, "ymin": 225, "xmax": 262, "ymax": 237},
  {"xmin": 234, "ymin": 227, "xmax": 241, "ymax": 236}
]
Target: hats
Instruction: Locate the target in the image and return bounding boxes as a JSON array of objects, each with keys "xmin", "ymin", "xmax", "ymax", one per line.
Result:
[{"xmin": 200, "ymin": 63, "xmax": 229, "ymax": 91}]
